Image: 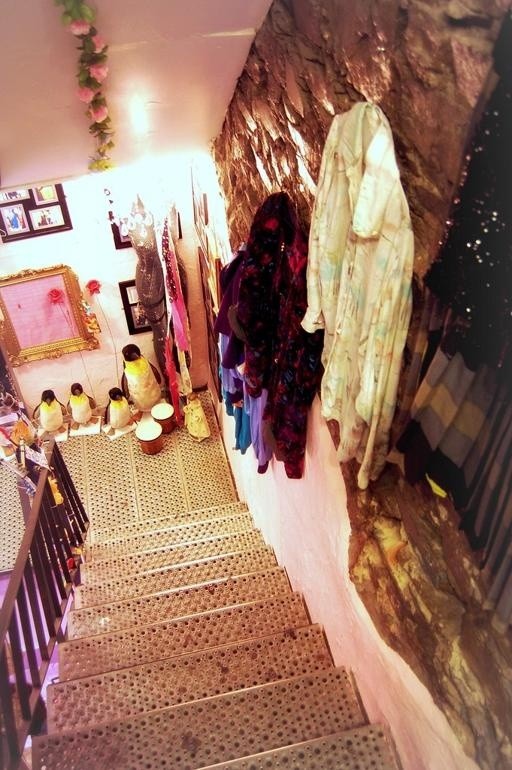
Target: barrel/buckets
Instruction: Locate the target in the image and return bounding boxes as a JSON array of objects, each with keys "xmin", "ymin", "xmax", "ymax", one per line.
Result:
[
  {"xmin": 151, "ymin": 403, "xmax": 175, "ymax": 433},
  {"xmin": 135, "ymin": 421, "xmax": 163, "ymax": 454}
]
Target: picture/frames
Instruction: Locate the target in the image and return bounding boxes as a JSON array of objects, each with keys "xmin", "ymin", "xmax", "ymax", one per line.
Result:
[
  {"xmin": 110, "ymin": 209, "xmax": 182, "ymax": 249},
  {"xmin": 192, "ymin": 190, "xmax": 225, "ymax": 403},
  {"xmin": 0, "ymin": 183, "xmax": 73, "ymax": 243},
  {"xmin": 0, "ymin": 264, "xmax": 101, "ymax": 368},
  {"xmin": 119, "ymin": 280, "xmax": 152, "ymax": 335}
]
